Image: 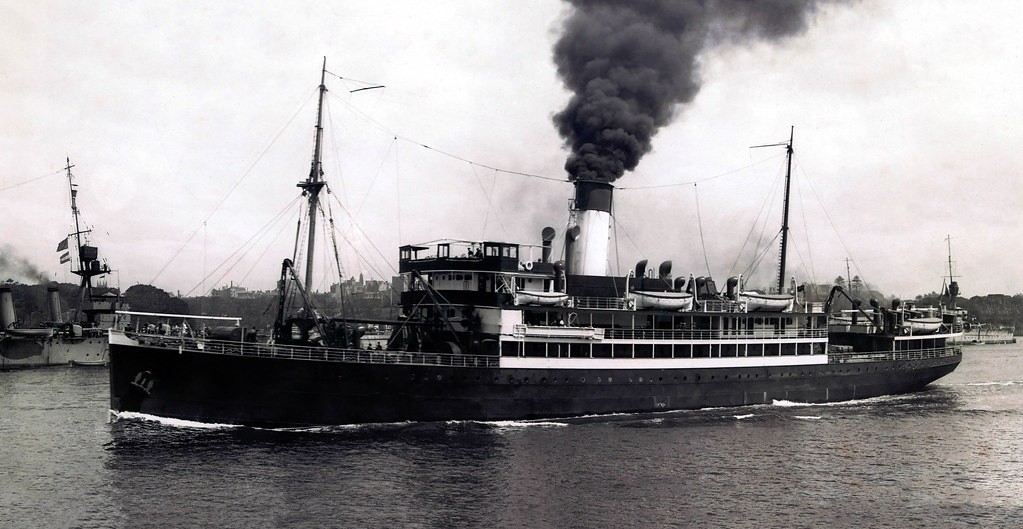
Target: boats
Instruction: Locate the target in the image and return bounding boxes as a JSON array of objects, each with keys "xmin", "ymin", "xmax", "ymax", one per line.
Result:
[
  {"xmin": 735, "ymin": 290, "xmax": 795, "ymax": 313},
  {"xmin": 623, "ymin": 290, "xmax": 694, "ymax": 312},
  {"xmin": 902, "ymin": 317, "xmax": 942, "ymax": 334},
  {"xmin": 104, "ymin": 55, "xmax": 966, "ymax": 432},
  {"xmin": 515, "ymin": 290, "xmax": 569, "ymax": 306}
]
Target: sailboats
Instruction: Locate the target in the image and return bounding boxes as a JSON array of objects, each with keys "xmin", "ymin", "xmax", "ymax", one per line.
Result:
[{"xmin": 0, "ymin": 155, "xmax": 140, "ymax": 369}]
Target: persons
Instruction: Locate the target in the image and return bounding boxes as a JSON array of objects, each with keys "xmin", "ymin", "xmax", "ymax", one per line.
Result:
[
  {"xmin": 360, "ymin": 344, "xmax": 366, "ymax": 350},
  {"xmin": 124, "ymin": 322, "xmax": 261, "ymax": 355},
  {"xmin": 467, "ymin": 248, "xmax": 473, "ymax": 258},
  {"xmin": 519, "ymin": 261, "xmax": 526, "ymax": 271},
  {"xmin": 366, "ymin": 344, "xmax": 374, "ymax": 353},
  {"xmin": 474, "ymin": 247, "xmax": 483, "ymax": 259},
  {"xmin": 375, "ymin": 341, "xmax": 382, "ymax": 350},
  {"xmin": 526, "ymin": 319, "xmax": 557, "ymax": 327}
]
